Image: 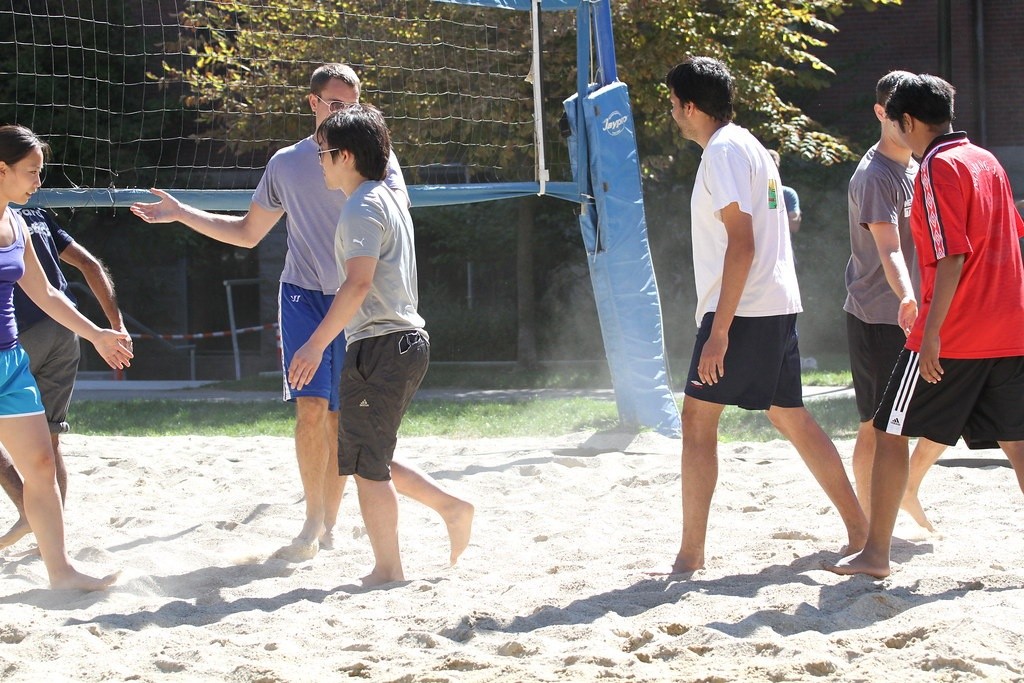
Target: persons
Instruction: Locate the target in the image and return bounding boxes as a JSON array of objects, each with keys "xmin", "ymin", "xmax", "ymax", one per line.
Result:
[
  {"xmin": 0, "ymin": 207, "xmax": 133, "ymax": 550},
  {"xmin": 843, "ymin": 71, "xmax": 948, "ymax": 532},
  {"xmin": 130, "ymin": 64, "xmax": 412, "ymax": 556},
  {"xmin": 818, "ymin": 75, "xmax": 1024, "ymax": 579},
  {"xmin": 766, "ymin": 149, "xmax": 802, "ymax": 266},
  {"xmin": 288, "ymin": 108, "xmax": 474, "ymax": 589},
  {"xmin": 642, "ymin": 58, "xmax": 869, "ymax": 575},
  {"xmin": 0, "ymin": 125, "xmax": 134, "ymax": 591}
]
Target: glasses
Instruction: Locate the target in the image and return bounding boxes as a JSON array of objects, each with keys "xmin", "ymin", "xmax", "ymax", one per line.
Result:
[
  {"xmin": 317, "ymin": 147, "xmax": 339, "ymax": 160},
  {"xmin": 315, "ymin": 95, "xmax": 361, "ymax": 115}
]
